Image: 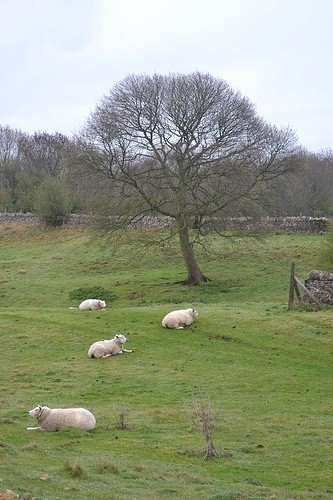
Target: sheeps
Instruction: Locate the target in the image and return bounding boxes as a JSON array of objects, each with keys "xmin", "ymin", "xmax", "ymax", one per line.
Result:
[
  {"xmin": 26, "ymin": 404, "xmax": 96, "ymax": 431},
  {"xmin": 161, "ymin": 308, "xmax": 199, "ymax": 330},
  {"xmin": 79, "ymin": 299, "xmax": 108, "ymax": 311},
  {"xmin": 88, "ymin": 333, "xmax": 135, "ymax": 359}
]
